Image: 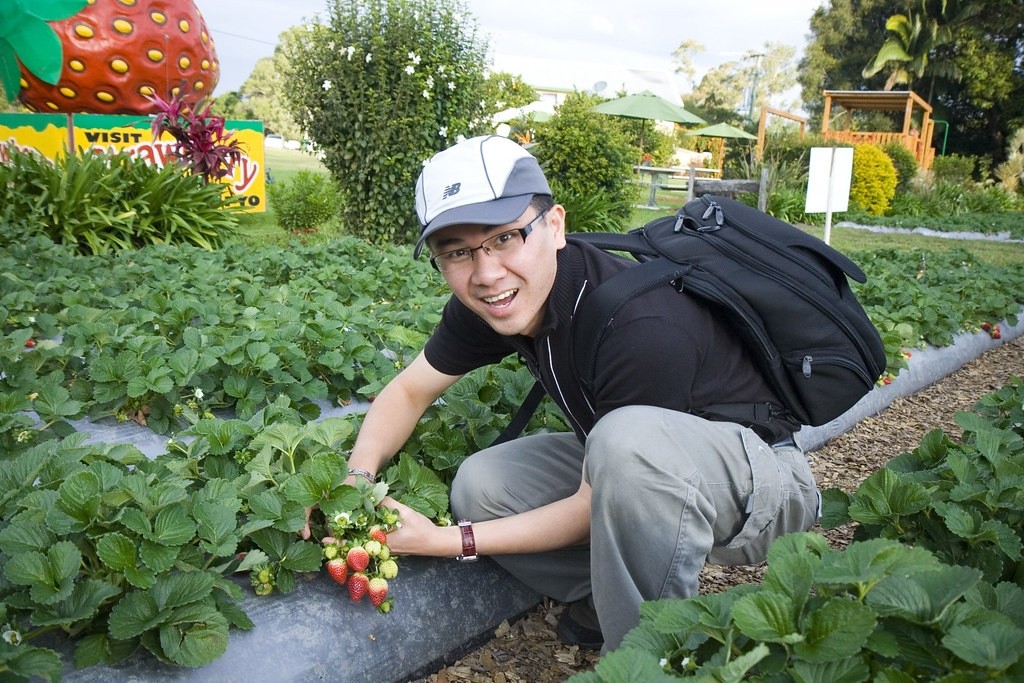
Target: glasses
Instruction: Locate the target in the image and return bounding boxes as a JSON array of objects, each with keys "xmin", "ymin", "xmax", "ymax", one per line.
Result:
[{"xmin": 429, "ymin": 207, "xmax": 549, "ymax": 273}]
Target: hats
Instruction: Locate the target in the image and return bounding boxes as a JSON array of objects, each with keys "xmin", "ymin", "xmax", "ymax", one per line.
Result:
[{"xmin": 414, "ymin": 133, "xmax": 552, "ymax": 260}]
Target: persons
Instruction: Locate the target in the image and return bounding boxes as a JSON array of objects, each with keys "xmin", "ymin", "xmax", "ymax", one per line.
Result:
[{"xmin": 300, "ymin": 136, "xmax": 823, "ymax": 658}]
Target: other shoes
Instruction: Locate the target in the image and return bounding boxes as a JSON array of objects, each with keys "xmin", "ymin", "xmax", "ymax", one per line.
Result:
[{"xmin": 557, "ymin": 608, "xmax": 604, "ymax": 650}]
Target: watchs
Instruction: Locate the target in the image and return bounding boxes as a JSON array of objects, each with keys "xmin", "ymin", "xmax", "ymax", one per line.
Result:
[{"xmin": 456, "ymin": 518, "xmax": 480, "ymax": 563}]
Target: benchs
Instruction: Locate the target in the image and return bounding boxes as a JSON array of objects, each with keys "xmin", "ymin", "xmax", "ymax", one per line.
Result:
[{"xmin": 628, "ymin": 180, "xmax": 689, "ymax": 191}]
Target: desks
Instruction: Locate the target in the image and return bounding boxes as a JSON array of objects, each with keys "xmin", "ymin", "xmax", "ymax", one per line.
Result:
[
  {"xmin": 633, "ymin": 165, "xmax": 677, "ymax": 210},
  {"xmin": 672, "ymin": 167, "xmax": 719, "ymax": 203}
]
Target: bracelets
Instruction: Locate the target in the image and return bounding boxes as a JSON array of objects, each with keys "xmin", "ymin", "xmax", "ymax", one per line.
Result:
[{"xmin": 348, "ymin": 469, "xmax": 376, "ymax": 484}]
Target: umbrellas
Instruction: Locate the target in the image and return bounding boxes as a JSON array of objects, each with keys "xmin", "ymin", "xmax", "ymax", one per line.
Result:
[
  {"xmin": 685, "ymin": 122, "xmax": 759, "ymax": 178},
  {"xmin": 595, "ymin": 90, "xmax": 707, "ymax": 174},
  {"xmin": 490, "ymin": 102, "xmax": 558, "ymax": 138}
]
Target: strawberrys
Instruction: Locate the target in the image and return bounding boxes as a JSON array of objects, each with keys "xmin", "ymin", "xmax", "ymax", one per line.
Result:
[
  {"xmin": 876, "ymin": 350, "xmax": 912, "ymax": 386},
  {"xmin": 254, "ymin": 570, "xmax": 273, "ymax": 595},
  {"xmin": 326, "ymin": 526, "xmax": 398, "ymax": 613},
  {"xmin": 971, "ymin": 322, "xmax": 1002, "ymax": 339},
  {"xmin": 25, "ymin": 338, "xmax": 36, "ymax": 348}
]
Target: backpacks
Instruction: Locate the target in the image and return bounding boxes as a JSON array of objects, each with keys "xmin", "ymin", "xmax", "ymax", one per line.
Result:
[{"xmin": 565, "ymin": 194, "xmax": 887, "ymax": 456}]
